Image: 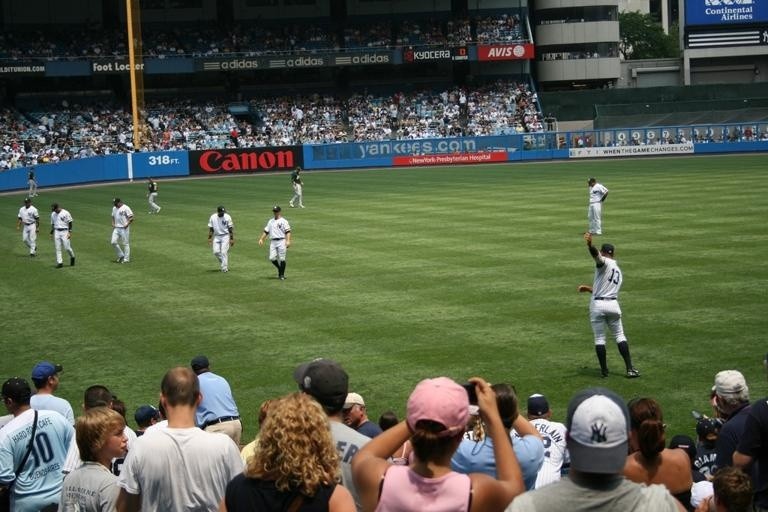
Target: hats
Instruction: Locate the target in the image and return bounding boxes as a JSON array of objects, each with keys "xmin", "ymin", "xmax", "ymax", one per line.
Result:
[
  {"xmin": 191, "ymin": 356, "xmax": 209, "ymax": 370},
  {"xmin": 669, "ymin": 435, "xmax": 696, "ymax": 459},
  {"xmin": 343, "ymin": 392, "xmax": 365, "ymax": 409},
  {"xmin": 24, "ymin": 166, "xmax": 302, "ymax": 213},
  {"xmin": 135, "ymin": 404, "xmax": 163, "ymax": 425},
  {"xmin": 294, "ymin": 358, "xmax": 348, "ymax": 399},
  {"xmin": 2, "ymin": 377, "xmax": 32, "ymax": 395},
  {"xmin": 31, "ymin": 361, "xmax": 63, "ymax": 379},
  {"xmin": 696, "ymin": 418, "xmax": 716, "ymax": 441},
  {"xmin": 566, "ymin": 388, "xmax": 631, "ymax": 474},
  {"xmin": 528, "ymin": 394, "xmax": 549, "ymax": 416},
  {"xmin": 711, "ymin": 370, "xmax": 745, "ymax": 393},
  {"xmin": 406, "ymin": 376, "xmax": 480, "ymax": 437},
  {"xmin": 600, "ymin": 244, "xmax": 614, "ymax": 254}
]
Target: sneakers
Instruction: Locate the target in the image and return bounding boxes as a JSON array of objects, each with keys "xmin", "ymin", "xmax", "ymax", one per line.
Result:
[
  {"xmin": 627, "ymin": 368, "xmax": 641, "ymax": 378},
  {"xmin": 31, "ymin": 204, "xmax": 305, "ymax": 280},
  {"xmin": 601, "ymin": 368, "xmax": 608, "ymax": 378}
]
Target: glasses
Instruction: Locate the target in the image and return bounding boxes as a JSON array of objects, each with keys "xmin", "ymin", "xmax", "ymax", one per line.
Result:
[{"xmin": 0, "ymin": 394, "xmax": 5, "ymax": 402}]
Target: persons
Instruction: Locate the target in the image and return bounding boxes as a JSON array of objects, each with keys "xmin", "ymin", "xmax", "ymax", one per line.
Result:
[
  {"xmin": 206, "ymin": 205, "xmax": 236, "ymax": 274},
  {"xmin": 16, "ymin": 198, "xmax": 40, "ymax": 257},
  {"xmin": 258, "ymin": 205, "xmax": 292, "ymax": 281},
  {"xmin": 0, "ymin": 9, "xmax": 768, "ymax": 174},
  {"xmin": 1, "ymin": 353, "xmax": 768, "ymax": 511},
  {"xmin": 577, "ymin": 233, "xmax": 641, "ymax": 378},
  {"xmin": 288, "ymin": 167, "xmax": 307, "ymax": 210},
  {"xmin": 583, "ymin": 176, "xmax": 610, "ymax": 239},
  {"xmin": 146, "ymin": 175, "xmax": 162, "ymax": 215},
  {"xmin": 109, "ymin": 197, "xmax": 135, "ymax": 263},
  {"xmin": 27, "ymin": 167, "xmax": 39, "ymax": 197},
  {"xmin": 49, "ymin": 202, "xmax": 75, "ymax": 269}
]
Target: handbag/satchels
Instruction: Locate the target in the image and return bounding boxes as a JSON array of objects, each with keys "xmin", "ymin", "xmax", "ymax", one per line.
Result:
[{"xmin": 0, "ymin": 483, "xmax": 10, "ymax": 512}]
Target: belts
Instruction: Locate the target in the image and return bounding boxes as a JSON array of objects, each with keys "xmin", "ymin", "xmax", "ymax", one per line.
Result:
[
  {"xmin": 202, "ymin": 417, "xmax": 238, "ymax": 430},
  {"xmin": 595, "ymin": 297, "xmax": 616, "ymax": 300},
  {"xmin": 25, "ymin": 222, "xmax": 35, "ymax": 226}
]
